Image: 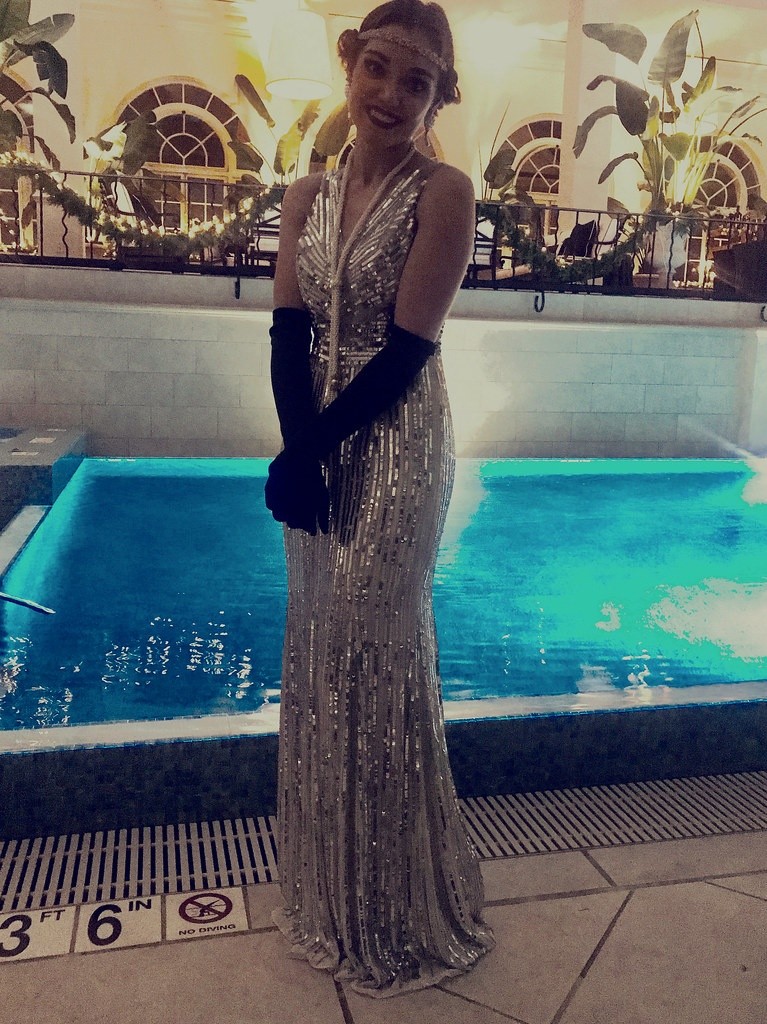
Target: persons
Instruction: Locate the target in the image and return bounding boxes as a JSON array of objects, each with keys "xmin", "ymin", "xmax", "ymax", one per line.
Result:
[{"xmin": 262, "ymin": 0, "xmax": 492, "ymax": 997}]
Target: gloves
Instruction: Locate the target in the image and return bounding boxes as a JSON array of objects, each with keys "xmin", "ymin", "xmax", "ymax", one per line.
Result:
[{"xmin": 265, "ymin": 306, "xmax": 436, "ymax": 535}]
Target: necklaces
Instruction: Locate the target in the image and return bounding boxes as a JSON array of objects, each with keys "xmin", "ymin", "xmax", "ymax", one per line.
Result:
[{"xmin": 323, "ymin": 141, "xmax": 416, "ymax": 410}]
